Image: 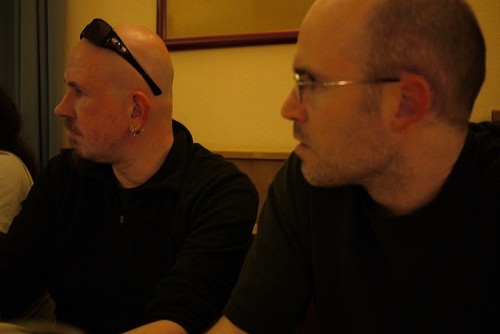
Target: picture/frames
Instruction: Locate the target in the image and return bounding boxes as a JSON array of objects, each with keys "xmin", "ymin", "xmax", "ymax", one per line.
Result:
[{"xmin": 156, "ymin": 0, "xmax": 318, "ymax": 51}]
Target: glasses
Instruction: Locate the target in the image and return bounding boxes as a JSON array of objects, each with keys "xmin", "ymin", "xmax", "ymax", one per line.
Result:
[
  {"xmin": 79, "ymin": 17, "xmax": 164, "ymax": 96},
  {"xmin": 292, "ymin": 74, "xmax": 402, "ymax": 103}
]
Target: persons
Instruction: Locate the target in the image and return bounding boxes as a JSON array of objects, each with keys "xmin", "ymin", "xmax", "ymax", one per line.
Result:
[
  {"xmin": 0, "ymin": 91, "xmax": 33, "ymax": 239},
  {"xmin": 0, "ymin": 23, "xmax": 259, "ymax": 334},
  {"xmin": 206, "ymin": 0, "xmax": 500, "ymax": 334}
]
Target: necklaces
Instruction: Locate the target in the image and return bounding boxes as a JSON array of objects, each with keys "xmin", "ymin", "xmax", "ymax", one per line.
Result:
[{"xmin": 115, "ymin": 162, "xmax": 155, "ymax": 187}]
